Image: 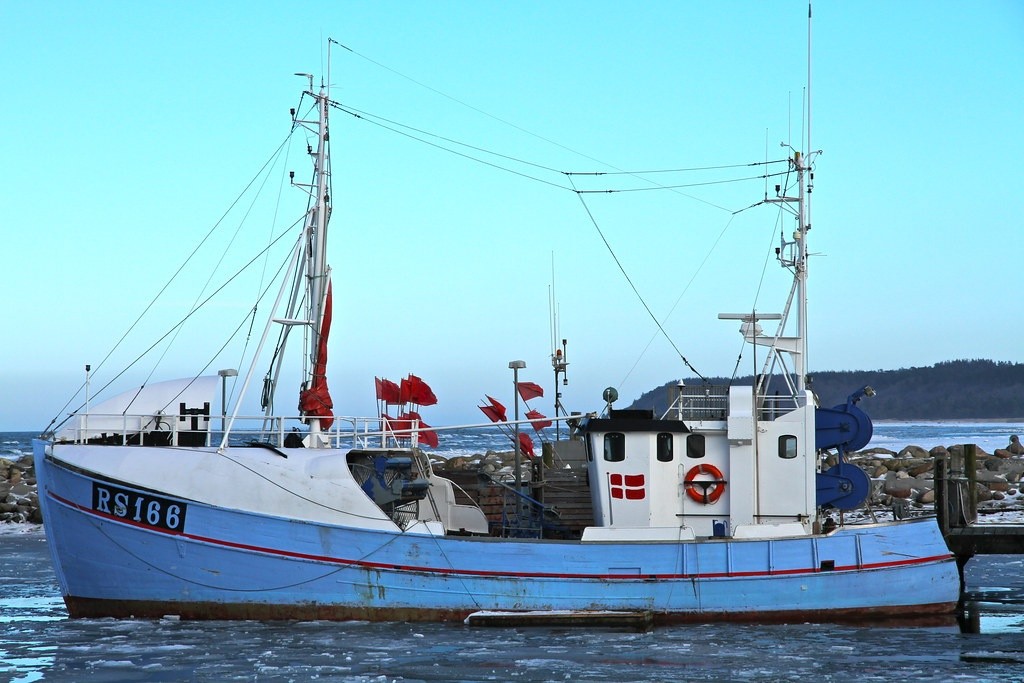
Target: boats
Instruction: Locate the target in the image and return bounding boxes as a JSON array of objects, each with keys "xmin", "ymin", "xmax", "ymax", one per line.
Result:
[{"xmin": 32, "ymin": 0, "xmax": 970, "ymax": 625}]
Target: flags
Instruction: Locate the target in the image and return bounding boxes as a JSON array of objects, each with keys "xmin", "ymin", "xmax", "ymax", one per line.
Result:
[
  {"xmin": 379, "ymin": 411, "xmax": 439, "ymax": 448},
  {"xmin": 515, "ymin": 381, "xmax": 544, "ymax": 403},
  {"xmin": 478, "ymin": 394, "xmax": 508, "ymax": 424},
  {"xmin": 523, "ymin": 410, "xmax": 552, "ymax": 430},
  {"xmin": 300, "ymin": 276, "xmax": 336, "ymax": 430},
  {"xmin": 371, "ymin": 375, "xmax": 438, "ymax": 410},
  {"xmin": 512, "ymin": 431, "xmax": 534, "ymax": 459}
]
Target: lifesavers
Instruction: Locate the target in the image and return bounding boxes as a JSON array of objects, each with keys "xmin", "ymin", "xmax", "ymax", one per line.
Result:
[{"xmin": 685, "ymin": 463, "xmax": 724, "ymax": 504}]
